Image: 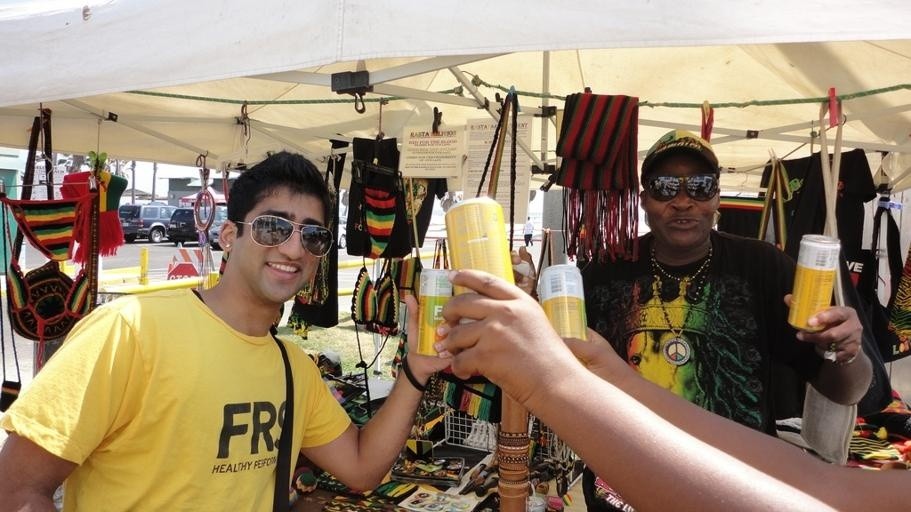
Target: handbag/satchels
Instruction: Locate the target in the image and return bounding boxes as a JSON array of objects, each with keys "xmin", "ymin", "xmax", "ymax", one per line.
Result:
[
  {"xmin": 8, "ymin": 259, "xmax": 78, "ymax": 342},
  {"xmin": 391, "ymin": 334, "xmax": 410, "ymax": 378},
  {"xmin": 441, "ymin": 373, "xmax": 502, "ymax": 425},
  {"xmin": 351, "ymin": 255, "xmax": 423, "ymax": 327},
  {"xmin": 800, "ymin": 284, "xmax": 911, "ymax": 466},
  {"xmin": 0, "ymin": 371, "xmax": 21, "ymax": 412}
]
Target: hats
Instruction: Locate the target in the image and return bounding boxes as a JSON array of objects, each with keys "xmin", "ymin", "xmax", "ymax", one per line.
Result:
[{"xmin": 641, "ymin": 129, "xmax": 718, "ymax": 170}]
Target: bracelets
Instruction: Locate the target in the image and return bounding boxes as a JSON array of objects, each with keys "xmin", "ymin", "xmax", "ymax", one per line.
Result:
[
  {"xmin": 832, "ymin": 344, "xmax": 862, "ymax": 367},
  {"xmin": 401, "ymin": 355, "xmax": 432, "ymax": 392}
]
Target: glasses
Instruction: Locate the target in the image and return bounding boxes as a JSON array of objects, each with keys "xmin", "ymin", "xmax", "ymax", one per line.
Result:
[
  {"xmin": 645, "ymin": 172, "xmax": 720, "ymax": 201},
  {"xmin": 234, "ymin": 215, "xmax": 336, "ymax": 258}
]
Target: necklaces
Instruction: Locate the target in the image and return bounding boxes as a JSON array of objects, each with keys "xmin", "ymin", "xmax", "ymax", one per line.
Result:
[
  {"xmin": 654, "ymin": 257, "xmax": 708, "ymax": 281},
  {"xmin": 651, "ymin": 241, "xmax": 713, "ymax": 365}
]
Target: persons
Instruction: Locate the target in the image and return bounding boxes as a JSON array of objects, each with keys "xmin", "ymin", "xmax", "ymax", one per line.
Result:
[
  {"xmin": 512, "ymin": 127, "xmax": 894, "ymax": 512},
  {"xmin": 523, "ymin": 217, "xmax": 535, "ymax": 246},
  {"xmin": 1, "ymin": 149, "xmax": 454, "ymax": 512},
  {"xmin": 441, "ymin": 266, "xmax": 910, "ymax": 512},
  {"xmin": 606, "ymin": 275, "xmax": 762, "ymax": 431}
]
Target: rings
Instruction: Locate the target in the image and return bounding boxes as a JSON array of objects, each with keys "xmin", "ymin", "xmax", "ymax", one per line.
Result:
[
  {"xmin": 829, "ymin": 342, "xmax": 836, "ymax": 352},
  {"xmin": 823, "ymin": 350, "xmax": 837, "ymax": 364}
]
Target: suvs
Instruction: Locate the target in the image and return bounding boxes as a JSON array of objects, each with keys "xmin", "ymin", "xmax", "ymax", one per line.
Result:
[
  {"xmin": 165, "ymin": 206, "xmax": 230, "ymax": 246},
  {"xmin": 118, "ymin": 201, "xmax": 180, "ymax": 243}
]
